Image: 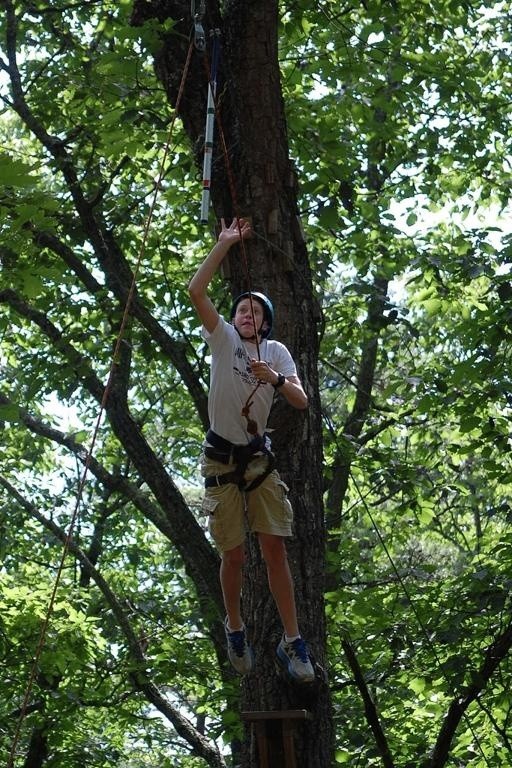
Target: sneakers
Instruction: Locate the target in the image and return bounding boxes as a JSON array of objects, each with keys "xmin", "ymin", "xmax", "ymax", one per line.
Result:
[
  {"xmin": 276, "ymin": 633, "xmax": 316, "ymax": 682},
  {"xmin": 224, "ymin": 615, "xmax": 252, "ymax": 674}
]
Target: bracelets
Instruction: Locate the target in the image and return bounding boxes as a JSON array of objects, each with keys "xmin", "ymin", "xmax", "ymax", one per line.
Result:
[{"xmin": 271, "ymin": 371, "xmax": 285, "ymax": 389}]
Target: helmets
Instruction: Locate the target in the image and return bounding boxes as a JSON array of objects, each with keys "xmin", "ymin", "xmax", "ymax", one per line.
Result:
[{"xmin": 230, "ymin": 293, "xmax": 274, "ymax": 341}]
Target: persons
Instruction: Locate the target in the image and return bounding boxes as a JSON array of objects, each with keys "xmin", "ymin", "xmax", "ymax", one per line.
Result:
[{"xmin": 186, "ymin": 215, "xmax": 316, "ymax": 686}]
